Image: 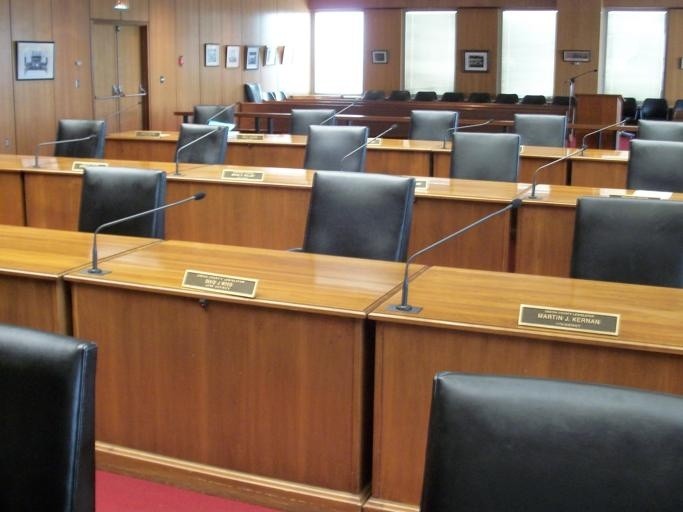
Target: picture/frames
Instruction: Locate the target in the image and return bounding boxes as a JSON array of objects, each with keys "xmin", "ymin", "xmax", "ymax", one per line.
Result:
[
  {"xmin": 205, "ymin": 43, "xmax": 277, "ymax": 70},
  {"xmin": 16, "ymin": 41, "xmax": 56, "ymax": 81},
  {"xmin": 464, "ymin": 50, "xmax": 488, "ymax": 72},
  {"xmin": 372, "ymin": 50, "xmax": 388, "ymax": 64},
  {"xmin": 564, "ymin": 50, "xmax": 591, "ymax": 62}
]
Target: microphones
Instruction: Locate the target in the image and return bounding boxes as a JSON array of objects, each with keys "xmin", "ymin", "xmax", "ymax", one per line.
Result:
[
  {"xmin": 571, "ymin": 69, "xmax": 598, "ymax": 80},
  {"xmin": 395, "ymin": 198, "xmax": 523, "ymax": 309},
  {"xmin": 442, "ymin": 119, "xmax": 495, "ymax": 150},
  {"xmin": 207, "ymin": 101, "xmax": 240, "ymax": 123},
  {"xmin": 107, "ymin": 101, "xmax": 142, "ymax": 118},
  {"xmin": 173, "ymin": 127, "xmax": 222, "ymax": 176},
  {"xmin": 86, "ymin": 192, "xmax": 206, "ymax": 274},
  {"xmin": 580, "ymin": 117, "xmax": 630, "ymax": 157},
  {"xmin": 528, "ymin": 144, "xmax": 588, "ymax": 198},
  {"xmin": 320, "ymin": 101, "xmax": 357, "ymax": 125},
  {"xmin": 32, "ymin": 135, "xmax": 97, "ymax": 168},
  {"xmin": 339, "ymin": 124, "xmax": 398, "ymax": 171}
]
